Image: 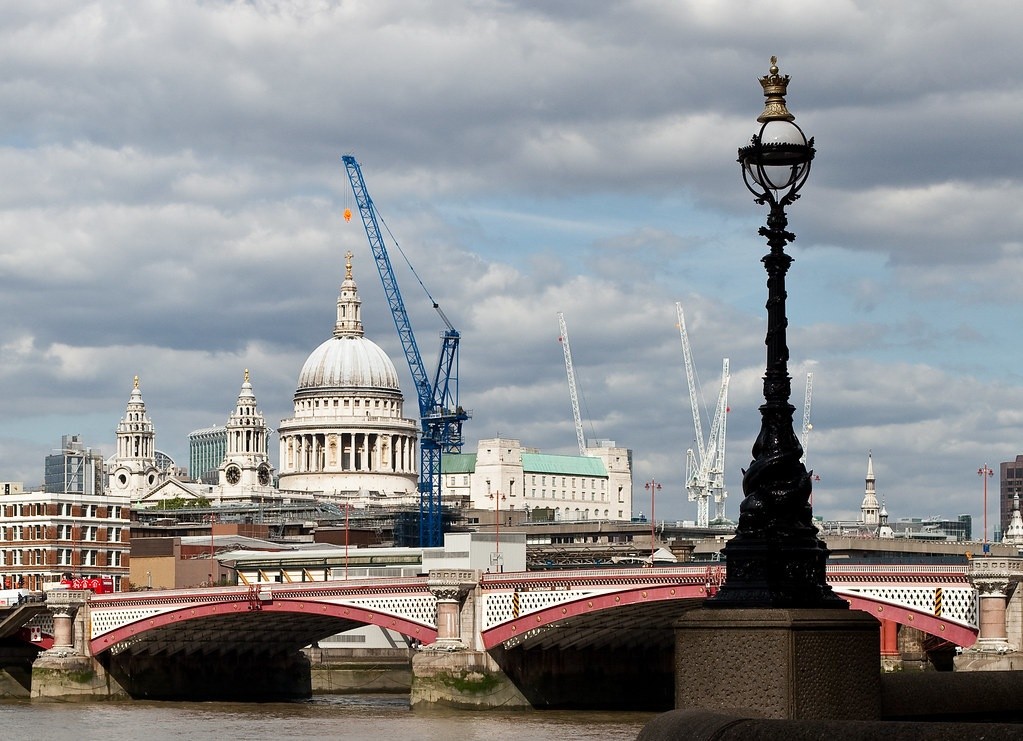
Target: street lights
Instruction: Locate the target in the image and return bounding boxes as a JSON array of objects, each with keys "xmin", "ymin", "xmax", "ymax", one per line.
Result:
[
  {"xmin": 977, "ymin": 463, "xmax": 994, "ymax": 543},
  {"xmin": 339, "ymin": 502, "xmax": 355, "ymax": 580},
  {"xmin": 203, "ymin": 512, "xmax": 219, "ymax": 587},
  {"xmin": 489, "ymin": 490, "xmax": 506, "ymax": 573},
  {"xmin": 645, "ymin": 478, "xmax": 662, "ymax": 567},
  {"xmin": 810, "ymin": 474, "xmax": 820, "ymax": 507}
]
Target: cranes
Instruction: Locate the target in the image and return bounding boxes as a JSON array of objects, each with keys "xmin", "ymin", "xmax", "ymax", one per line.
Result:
[
  {"xmin": 800, "ymin": 373, "xmax": 814, "ymax": 468},
  {"xmin": 557, "ymin": 312, "xmax": 599, "ymax": 457},
  {"xmin": 342, "ymin": 154, "xmax": 469, "ymax": 546},
  {"xmin": 675, "ymin": 302, "xmax": 731, "ymax": 529}
]
